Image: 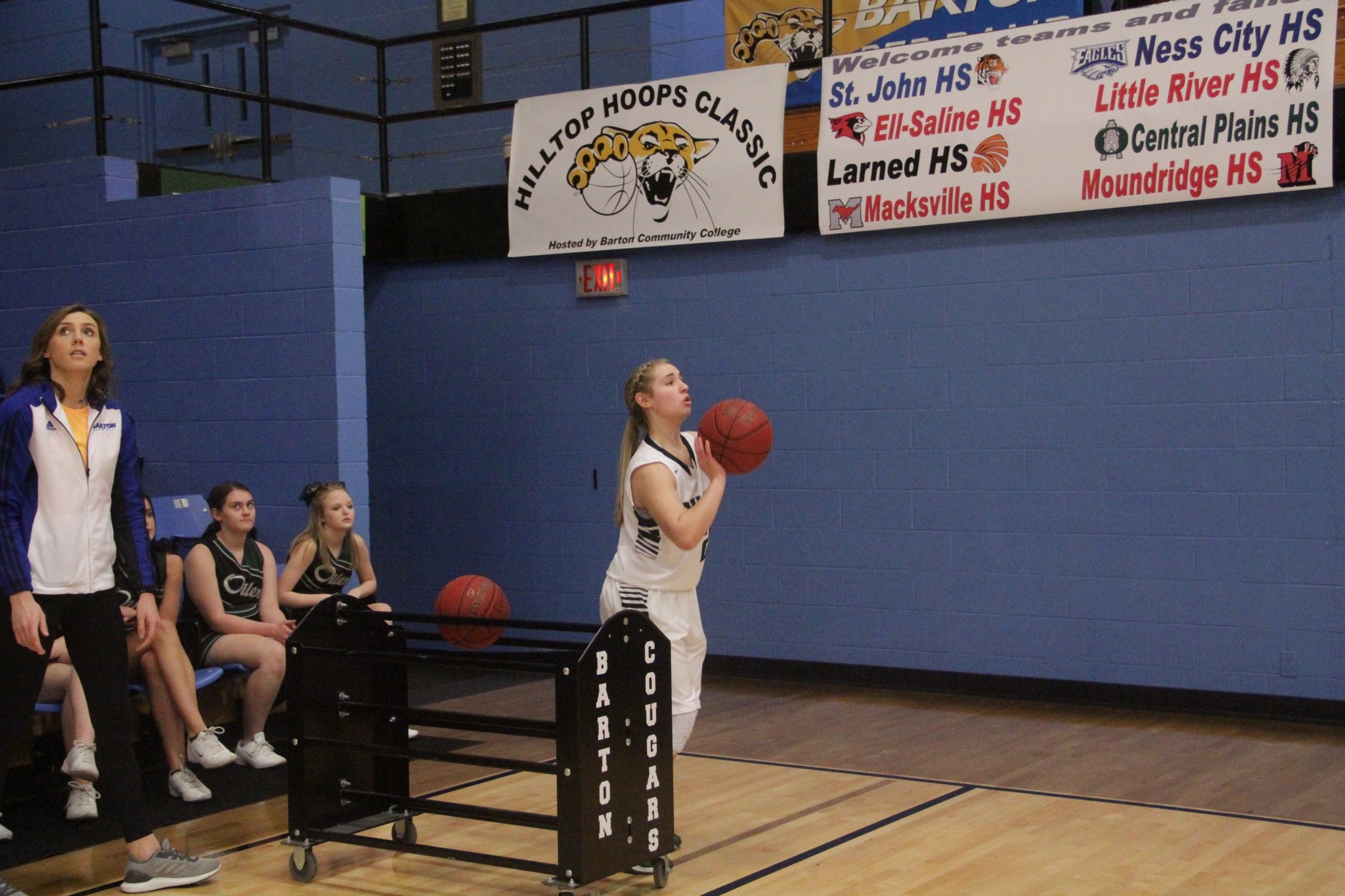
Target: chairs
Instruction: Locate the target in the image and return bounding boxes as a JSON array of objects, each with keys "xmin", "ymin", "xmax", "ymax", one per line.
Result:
[{"xmin": 32, "ymin": 494, "xmax": 300, "ymax": 712}]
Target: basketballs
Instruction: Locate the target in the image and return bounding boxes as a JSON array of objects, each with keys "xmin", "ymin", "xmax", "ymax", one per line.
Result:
[
  {"xmin": 697, "ymin": 397, "xmax": 774, "ymax": 475},
  {"xmin": 434, "ymin": 573, "xmax": 511, "ymax": 651}
]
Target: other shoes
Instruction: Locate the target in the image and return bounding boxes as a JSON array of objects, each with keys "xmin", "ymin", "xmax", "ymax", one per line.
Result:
[
  {"xmin": 0, "ymin": 824, "xmax": 12, "ymax": 841},
  {"xmin": 626, "ymin": 857, "xmax": 675, "ymax": 876}
]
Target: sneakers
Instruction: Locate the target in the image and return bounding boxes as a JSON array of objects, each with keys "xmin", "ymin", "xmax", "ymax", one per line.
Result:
[
  {"xmin": 60, "ymin": 740, "xmax": 100, "ymax": 780},
  {"xmin": 186, "ymin": 727, "xmax": 238, "ymax": 769},
  {"xmin": 167, "ymin": 754, "xmax": 214, "ymax": 802},
  {"xmin": 234, "ymin": 731, "xmax": 287, "ymax": 771},
  {"xmin": 120, "ymin": 839, "xmax": 221, "ymax": 893},
  {"xmin": 0, "ymin": 879, "xmax": 27, "ymax": 896},
  {"xmin": 64, "ymin": 781, "xmax": 101, "ymax": 822}
]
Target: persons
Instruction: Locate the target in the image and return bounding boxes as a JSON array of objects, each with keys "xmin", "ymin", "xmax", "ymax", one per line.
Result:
[
  {"xmin": 277, "ymin": 481, "xmax": 419, "ymax": 739},
  {"xmin": 115, "ymin": 495, "xmax": 237, "ymax": 801},
  {"xmin": 0, "ymin": 303, "xmax": 221, "ymax": 896},
  {"xmin": 178, "ymin": 482, "xmax": 296, "ymax": 768},
  {"xmin": 38, "ymin": 635, "xmax": 100, "ymax": 819},
  {"xmin": 599, "ymin": 358, "xmax": 726, "ymax": 758}
]
